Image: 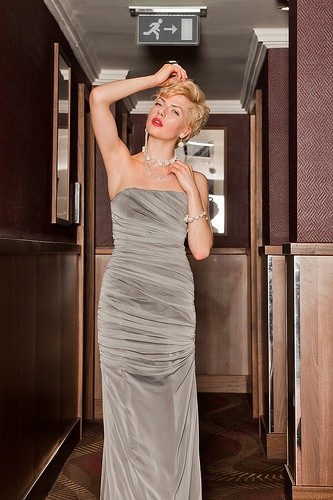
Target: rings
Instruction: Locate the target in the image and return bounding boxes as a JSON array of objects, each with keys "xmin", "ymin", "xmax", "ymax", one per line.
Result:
[{"xmin": 169, "ymin": 61, "xmax": 176, "ymax": 64}]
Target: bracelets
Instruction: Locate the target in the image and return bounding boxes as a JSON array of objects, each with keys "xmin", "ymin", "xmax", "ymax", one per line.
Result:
[{"xmin": 184, "ymin": 211, "xmax": 209, "ymax": 223}]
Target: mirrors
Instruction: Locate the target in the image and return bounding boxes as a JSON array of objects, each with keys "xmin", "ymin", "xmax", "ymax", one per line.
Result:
[{"xmin": 53, "ymin": 42, "xmax": 72, "ymax": 225}]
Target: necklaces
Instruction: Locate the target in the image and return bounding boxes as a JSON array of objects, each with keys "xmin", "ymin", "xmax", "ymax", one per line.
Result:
[{"xmin": 142, "ymin": 146, "xmax": 177, "ymax": 166}]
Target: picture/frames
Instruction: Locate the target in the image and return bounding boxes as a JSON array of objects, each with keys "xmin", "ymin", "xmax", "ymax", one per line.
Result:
[{"xmin": 144, "ymin": 125, "xmax": 229, "ymax": 236}]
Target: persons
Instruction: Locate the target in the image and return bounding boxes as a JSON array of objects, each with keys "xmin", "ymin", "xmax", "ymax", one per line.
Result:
[{"xmin": 88, "ymin": 61, "xmax": 212, "ymax": 500}]
